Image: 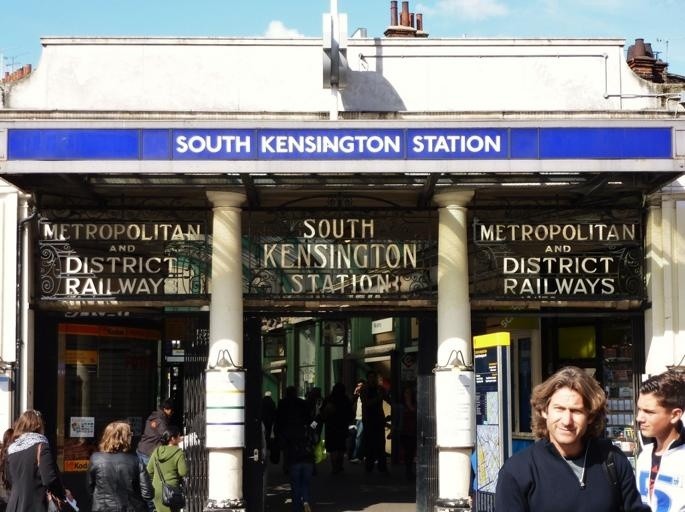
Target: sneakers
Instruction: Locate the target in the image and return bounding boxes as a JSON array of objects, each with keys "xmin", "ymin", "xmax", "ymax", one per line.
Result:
[
  {"xmin": 266, "ymin": 487, "xmax": 293, "ymax": 497},
  {"xmin": 303, "ymin": 500, "xmax": 312, "ymax": 511},
  {"xmin": 345, "ymin": 454, "xmax": 379, "ymax": 465}
]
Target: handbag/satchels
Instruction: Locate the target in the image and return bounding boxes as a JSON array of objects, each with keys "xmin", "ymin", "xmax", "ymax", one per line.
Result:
[
  {"xmin": 46, "ymin": 492, "xmax": 75, "ymax": 512},
  {"xmin": 162, "ymin": 483, "xmax": 186, "ymax": 508}
]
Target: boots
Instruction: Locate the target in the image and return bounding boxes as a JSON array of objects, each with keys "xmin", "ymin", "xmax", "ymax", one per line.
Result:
[{"xmin": 331, "ymin": 457, "xmax": 344, "ymax": 474}]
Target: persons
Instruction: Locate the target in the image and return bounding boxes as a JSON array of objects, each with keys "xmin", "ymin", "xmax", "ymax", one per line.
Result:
[
  {"xmin": 0, "ymin": 410, "xmax": 65, "ymax": 512},
  {"xmin": 260, "ymin": 371, "xmax": 391, "ymax": 512},
  {"xmin": 636, "ymin": 370, "xmax": 685, "ymax": 511},
  {"xmin": 495, "ymin": 365, "xmax": 651, "ymax": 511},
  {"xmin": 88, "ymin": 397, "xmax": 188, "ymax": 512}
]
